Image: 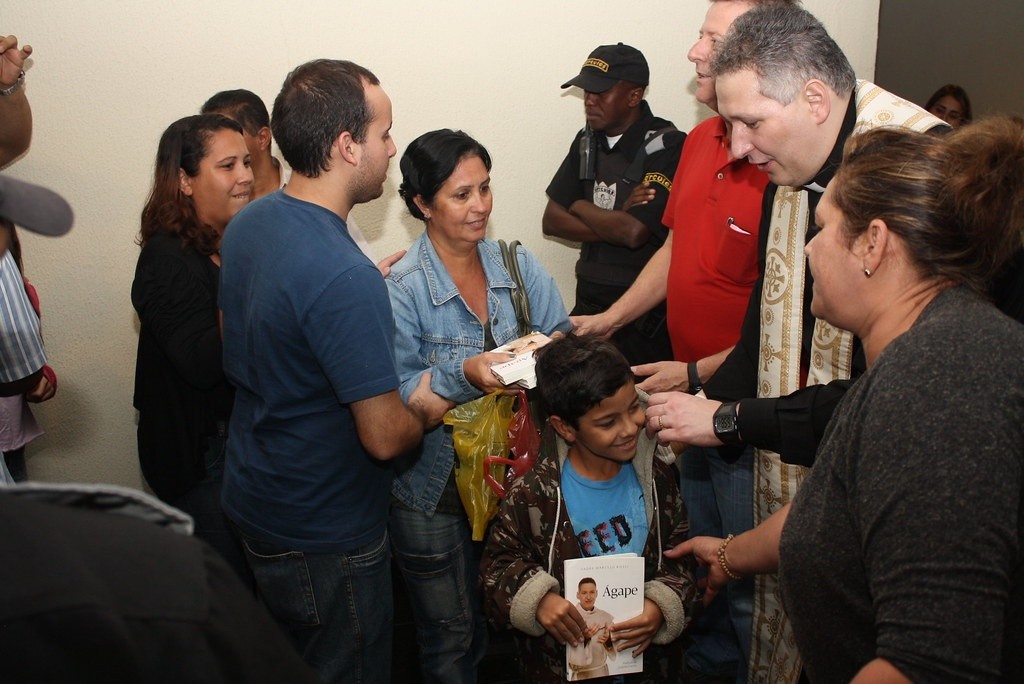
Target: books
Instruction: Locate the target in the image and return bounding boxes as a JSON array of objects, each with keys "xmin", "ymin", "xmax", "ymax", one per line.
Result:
[
  {"xmin": 486, "ymin": 331, "xmax": 553, "ymax": 389},
  {"xmin": 563, "ymin": 553, "xmax": 643, "ymax": 681}
]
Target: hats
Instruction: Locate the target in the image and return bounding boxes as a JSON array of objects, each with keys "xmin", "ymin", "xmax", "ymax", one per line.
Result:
[{"xmin": 561, "ymin": 42, "xmax": 650, "ymax": 93}]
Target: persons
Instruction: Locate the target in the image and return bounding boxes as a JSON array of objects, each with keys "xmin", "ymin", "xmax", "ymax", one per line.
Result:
[
  {"xmin": 568, "ymin": 578, "xmax": 618, "ymax": 681},
  {"xmin": 200, "ymin": 88, "xmax": 293, "ymax": 201},
  {"xmin": 661, "ymin": 115, "xmax": 1023, "ymax": 684},
  {"xmin": 567, "ymin": 1, "xmax": 773, "ymax": 684},
  {"xmin": 924, "ymin": 84, "xmax": 972, "ymax": 131},
  {"xmin": 540, "ymin": 42, "xmax": 688, "ymax": 384},
  {"xmin": 647, "ymin": 0, "xmax": 955, "ymax": 684},
  {"xmin": 380, "ymin": 130, "xmax": 572, "ymax": 684},
  {"xmin": 132, "ymin": 115, "xmax": 256, "ymax": 602},
  {"xmin": 215, "ymin": 59, "xmax": 424, "ymax": 684},
  {"xmin": 476, "ymin": 332, "xmax": 701, "ymax": 684},
  {"xmin": 0, "ymin": 33, "xmax": 319, "ymax": 684},
  {"xmin": 503, "ymin": 333, "xmax": 552, "ymax": 355}
]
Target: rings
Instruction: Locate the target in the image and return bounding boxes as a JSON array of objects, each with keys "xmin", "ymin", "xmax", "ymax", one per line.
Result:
[{"xmin": 658, "ymin": 415, "xmax": 665, "ymax": 428}]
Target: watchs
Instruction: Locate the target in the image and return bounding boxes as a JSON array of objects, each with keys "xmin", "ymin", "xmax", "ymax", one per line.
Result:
[{"xmin": 713, "ymin": 400, "xmax": 742, "ymax": 447}]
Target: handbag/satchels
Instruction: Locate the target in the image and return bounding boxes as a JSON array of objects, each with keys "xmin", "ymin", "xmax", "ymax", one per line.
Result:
[
  {"xmin": 484, "ymin": 392, "xmax": 539, "ymax": 498},
  {"xmin": 443, "ymin": 384, "xmax": 517, "ymax": 543}
]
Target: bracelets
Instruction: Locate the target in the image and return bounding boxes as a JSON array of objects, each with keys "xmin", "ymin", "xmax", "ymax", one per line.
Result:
[
  {"xmin": 718, "ymin": 534, "xmax": 744, "ymax": 581},
  {"xmin": 0, "ymin": 70, "xmax": 25, "ymax": 96},
  {"xmin": 686, "ymin": 359, "xmax": 703, "ymax": 394}
]
